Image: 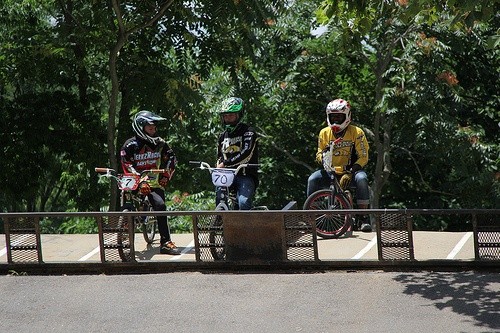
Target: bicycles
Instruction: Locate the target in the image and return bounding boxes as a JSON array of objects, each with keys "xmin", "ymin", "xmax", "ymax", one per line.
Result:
[
  {"xmin": 95, "ymin": 167, "xmax": 169, "ymax": 262},
  {"xmin": 188, "ymin": 160, "xmax": 269, "ymax": 261},
  {"xmin": 302, "ymin": 137, "xmax": 365, "ymax": 239}
]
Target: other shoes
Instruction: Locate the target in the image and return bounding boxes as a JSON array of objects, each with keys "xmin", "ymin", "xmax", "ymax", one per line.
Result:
[
  {"xmin": 118, "ymin": 240, "xmax": 132, "ymax": 249},
  {"xmin": 160, "ymin": 241, "xmax": 181, "ymax": 255},
  {"xmin": 359, "ymin": 214, "xmax": 372, "ymax": 232}
]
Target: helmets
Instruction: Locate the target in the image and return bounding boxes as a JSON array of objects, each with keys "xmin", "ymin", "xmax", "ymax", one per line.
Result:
[
  {"xmin": 326, "ymin": 98, "xmax": 351, "ymax": 133},
  {"xmin": 219, "ymin": 97, "xmax": 248, "ymax": 133},
  {"xmin": 131, "ymin": 110, "xmax": 167, "ymax": 150}
]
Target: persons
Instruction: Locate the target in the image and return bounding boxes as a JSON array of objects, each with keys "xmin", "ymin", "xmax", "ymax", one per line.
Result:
[
  {"xmin": 299, "ymin": 99, "xmax": 371, "ymax": 233},
  {"xmin": 120, "ymin": 111, "xmax": 181, "ymax": 254},
  {"xmin": 216, "ymin": 97, "xmax": 259, "ymax": 210}
]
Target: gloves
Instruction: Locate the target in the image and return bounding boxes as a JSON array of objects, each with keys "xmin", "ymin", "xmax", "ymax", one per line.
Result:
[
  {"xmin": 316, "ymin": 153, "xmax": 323, "ymax": 165},
  {"xmin": 139, "ymin": 181, "xmax": 151, "ymax": 195},
  {"xmin": 340, "ymin": 173, "xmax": 353, "ymax": 190}
]
